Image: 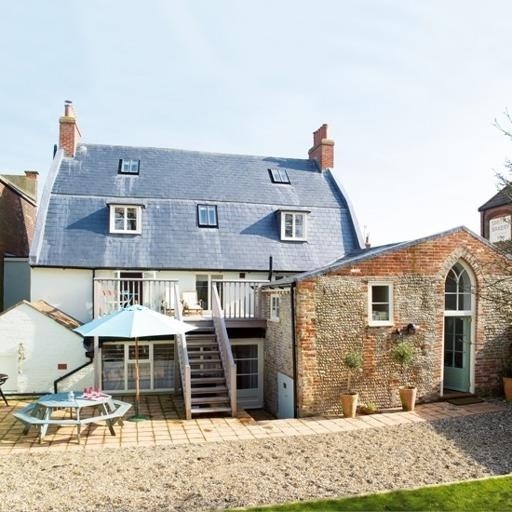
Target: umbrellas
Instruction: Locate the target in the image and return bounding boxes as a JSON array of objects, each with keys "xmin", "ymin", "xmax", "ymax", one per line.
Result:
[{"xmin": 73, "ymin": 303, "xmax": 199, "ymax": 425}]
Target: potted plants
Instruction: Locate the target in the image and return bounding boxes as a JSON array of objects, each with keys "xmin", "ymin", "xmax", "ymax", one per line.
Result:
[{"xmin": 339, "ymin": 351, "xmax": 364, "ymax": 419}]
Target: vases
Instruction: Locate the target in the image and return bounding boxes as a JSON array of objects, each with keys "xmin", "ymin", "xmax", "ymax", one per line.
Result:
[
  {"xmin": 499, "ymin": 376, "xmax": 511, "ymax": 401},
  {"xmin": 389, "ymin": 342, "xmax": 418, "ymax": 412}
]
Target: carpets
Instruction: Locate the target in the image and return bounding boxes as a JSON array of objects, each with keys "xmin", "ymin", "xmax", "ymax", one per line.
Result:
[{"xmin": 446, "ymin": 394, "xmax": 484, "ymax": 406}]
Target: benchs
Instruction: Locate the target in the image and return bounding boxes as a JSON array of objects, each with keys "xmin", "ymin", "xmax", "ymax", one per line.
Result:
[{"xmin": 1, "ymin": 370, "xmax": 137, "ymax": 444}]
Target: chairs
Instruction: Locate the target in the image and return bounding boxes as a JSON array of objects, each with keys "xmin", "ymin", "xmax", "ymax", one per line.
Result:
[
  {"xmin": 101, "ymin": 289, "xmax": 131, "ymax": 313},
  {"xmin": 181, "ymin": 290, "xmax": 203, "ymax": 316},
  {"xmin": 162, "ymin": 286, "xmax": 184, "ymax": 315}
]
